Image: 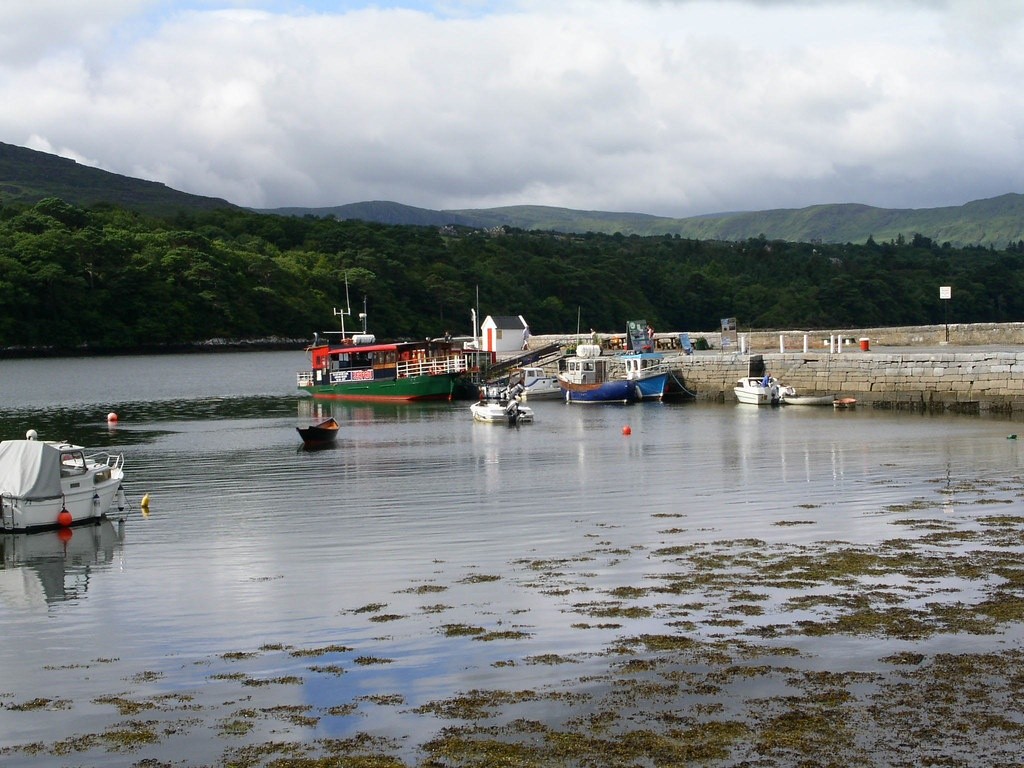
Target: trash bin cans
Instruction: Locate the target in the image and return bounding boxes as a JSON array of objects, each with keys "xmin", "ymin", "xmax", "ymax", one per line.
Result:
[{"xmin": 860, "ymin": 337, "xmax": 869, "ymax": 351}]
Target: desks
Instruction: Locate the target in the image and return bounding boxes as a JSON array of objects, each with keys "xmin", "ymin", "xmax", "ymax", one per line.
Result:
[{"xmin": 608, "ymin": 337, "xmax": 676, "ymax": 349}]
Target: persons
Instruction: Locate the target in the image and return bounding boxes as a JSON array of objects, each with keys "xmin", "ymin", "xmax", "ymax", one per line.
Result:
[
  {"xmin": 591, "ymin": 329, "xmax": 596, "ymax": 339},
  {"xmin": 647, "ymin": 325, "xmax": 654, "ymax": 342},
  {"xmin": 521, "ymin": 325, "xmax": 532, "ymax": 351},
  {"xmin": 444, "ymin": 331, "xmax": 452, "ymax": 343}
]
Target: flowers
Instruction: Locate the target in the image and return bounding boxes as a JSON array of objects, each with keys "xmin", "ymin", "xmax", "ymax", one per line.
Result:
[{"xmin": 642, "ymin": 345, "xmax": 651, "ymax": 349}]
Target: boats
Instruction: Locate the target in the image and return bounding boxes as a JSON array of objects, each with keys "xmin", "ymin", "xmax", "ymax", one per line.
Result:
[
  {"xmin": 553, "ymin": 305, "xmax": 635, "ymax": 406},
  {"xmin": 296, "ymin": 417, "xmax": 341, "ymax": 446},
  {"xmin": 295, "ymin": 271, "xmax": 481, "ymax": 402},
  {"xmin": 831, "ymin": 397, "xmax": 858, "ymax": 409},
  {"xmin": 469, "ymin": 385, "xmax": 535, "ymax": 424},
  {"xmin": 782, "ymin": 393, "xmax": 835, "ymax": 406},
  {"xmin": 611, "ymin": 351, "xmax": 687, "ymax": 404},
  {"xmin": 0, "ymin": 432, "xmax": 124, "ymax": 533},
  {"xmin": 476, "ymin": 362, "xmax": 563, "ymax": 401},
  {"xmin": 732, "ymin": 328, "xmax": 796, "ymax": 406}
]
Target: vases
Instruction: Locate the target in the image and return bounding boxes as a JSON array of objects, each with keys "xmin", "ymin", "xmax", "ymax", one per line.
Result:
[{"xmin": 643, "ymin": 348, "xmax": 651, "ymax": 353}]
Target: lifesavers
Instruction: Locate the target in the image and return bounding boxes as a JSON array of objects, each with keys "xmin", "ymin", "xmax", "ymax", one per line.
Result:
[{"xmin": 429, "ymin": 365, "xmax": 442, "ymax": 375}]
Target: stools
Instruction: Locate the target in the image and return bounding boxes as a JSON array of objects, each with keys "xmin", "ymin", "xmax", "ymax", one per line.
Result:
[{"xmin": 659, "ymin": 342, "xmax": 668, "ymax": 349}]
